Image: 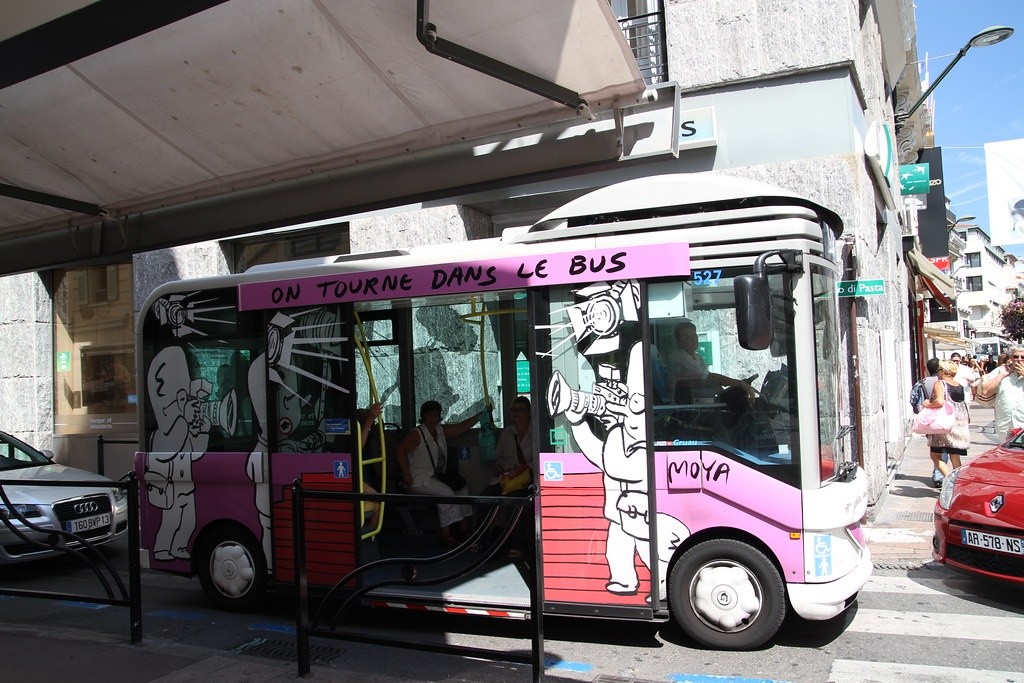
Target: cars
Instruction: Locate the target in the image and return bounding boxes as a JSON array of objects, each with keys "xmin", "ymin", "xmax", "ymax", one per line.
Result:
[
  {"xmin": 930, "ymin": 425, "xmax": 1024, "ymax": 589},
  {"xmin": 0, "ymin": 429, "xmax": 126, "ymax": 563}
]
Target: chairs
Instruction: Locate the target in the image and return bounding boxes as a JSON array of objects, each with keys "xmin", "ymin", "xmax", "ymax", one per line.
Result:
[
  {"xmin": 647, "ymin": 343, "xmax": 727, "ymax": 436},
  {"xmin": 356, "ymin": 420, "xmax": 432, "ymax": 532}
]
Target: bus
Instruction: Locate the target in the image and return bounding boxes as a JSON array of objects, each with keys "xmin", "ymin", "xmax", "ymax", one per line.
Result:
[{"xmin": 125, "ymin": 171, "xmax": 868, "ymax": 655}]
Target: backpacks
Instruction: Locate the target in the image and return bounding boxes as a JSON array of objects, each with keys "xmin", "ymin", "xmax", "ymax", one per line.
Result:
[{"xmin": 913, "ymin": 386, "xmax": 924, "ymax": 414}]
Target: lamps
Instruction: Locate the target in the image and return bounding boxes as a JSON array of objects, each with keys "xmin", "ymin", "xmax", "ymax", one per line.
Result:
[
  {"xmin": 956, "ymin": 289, "xmax": 968, "ymax": 297},
  {"xmin": 948, "ymin": 215, "xmax": 977, "ymax": 231},
  {"xmin": 950, "ymin": 264, "xmax": 969, "ymax": 278},
  {"xmin": 893, "ymin": 25, "xmax": 1014, "ymax": 128}
]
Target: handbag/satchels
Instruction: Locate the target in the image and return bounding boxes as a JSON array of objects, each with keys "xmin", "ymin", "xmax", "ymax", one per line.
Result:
[
  {"xmin": 439, "ymin": 471, "xmax": 467, "ymax": 491},
  {"xmin": 501, "ymin": 465, "xmax": 532, "ymax": 494},
  {"xmin": 913, "ymin": 401, "xmax": 955, "ymax": 434}
]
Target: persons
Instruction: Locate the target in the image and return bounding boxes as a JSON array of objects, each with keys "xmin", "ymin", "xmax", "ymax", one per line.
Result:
[
  {"xmin": 981, "ymin": 345, "xmax": 1024, "ymax": 443},
  {"xmin": 99, "ymin": 357, "xmax": 130, "ymax": 401},
  {"xmin": 951, "ymin": 352, "xmax": 976, "ymax": 408},
  {"xmin": 960, "ymin": 353, "xmax": 978, "ymax": 367},
  {"xmin": 910, "ymin": 358, "xmax": 949, "ymax": 486},
  {"xmin": 398, "ymin": 401, "xmax": 493, "ymax": 544},
  {"xmin": 983, "ymin": 354, "xmax": 996, "ymax": 374},
  {"xmin": 920, "ymin": 361, "xmax": 966, "ymax": 478},
  {"xmin": 468, "ymin": 396, "xmax": 533, "ymax": 558},
  {"xmin": 667, "ymin": 321, "xmax": 760, "ymax": 405},
  {"xmin": 968, "ymin": 357, "xmax": 979, "ymax": 400},
  {"xmin": 361, "ymin": 403, "xmax": 383, "ymax": 542}
]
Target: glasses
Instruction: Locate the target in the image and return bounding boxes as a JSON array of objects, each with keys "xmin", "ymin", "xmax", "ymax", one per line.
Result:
[
  {"xmin": 1012, "ymin": 355, "xmax": 1024, "ymax": 360},
  {"xmin": 509, "ymin": 407, "xmax": 529, "ymax": 413},
  {"xmin": 952, "ymin": 360, "xmax": 960, "ymax": 362}
]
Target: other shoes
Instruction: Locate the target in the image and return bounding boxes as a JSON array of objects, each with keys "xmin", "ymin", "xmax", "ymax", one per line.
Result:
[
  {"xmin": 935, "ymin": 481, "xmax": 942, "ymax": 487},
  {"xmin": 444, "ymin": 530, "xmax": 479, "ymax": 545}
]
